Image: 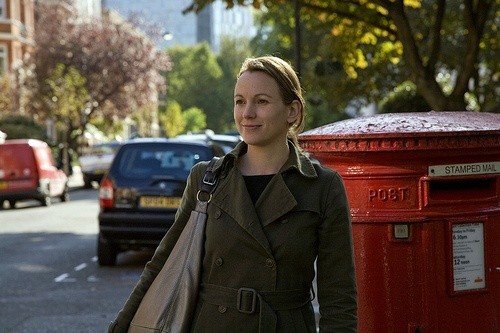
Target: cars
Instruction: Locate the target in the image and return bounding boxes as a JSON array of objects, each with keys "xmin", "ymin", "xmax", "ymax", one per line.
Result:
[
  {"xmin": 96, "ymin": 136, "xmax": 227, "ymax": 268},
  {"xmin": 78, "ymin": 143, "xmax": 119, "ymax": 190},
  {"xmin": 0, "ymin": 138, "xmax": 70, "ymax": 206}
]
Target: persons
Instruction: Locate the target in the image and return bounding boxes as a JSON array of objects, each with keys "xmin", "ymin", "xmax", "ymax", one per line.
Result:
[{"xmin": 108, "ymin": 56, "xmax": 357, "ymax": 333}]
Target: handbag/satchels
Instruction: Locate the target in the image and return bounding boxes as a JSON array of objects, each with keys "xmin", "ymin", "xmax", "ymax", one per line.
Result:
[{"xmin": 127, "ymin": 202, "xmax": 207, "ymax": 333}]
